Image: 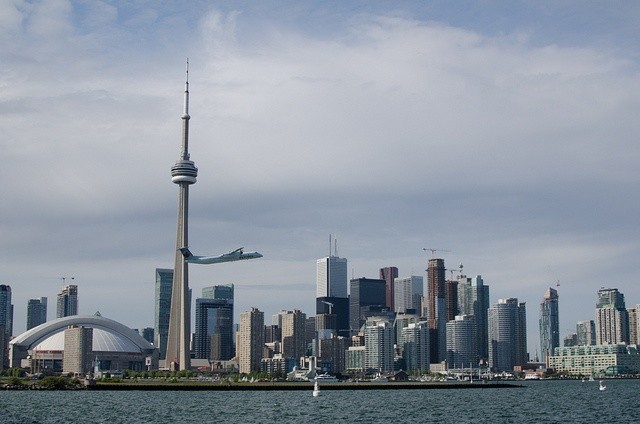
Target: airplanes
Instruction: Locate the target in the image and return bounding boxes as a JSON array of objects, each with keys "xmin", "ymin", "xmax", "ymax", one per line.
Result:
[{"xmin": 177, "ymin": 247, "xmax": 264, "ymax": 265}]
